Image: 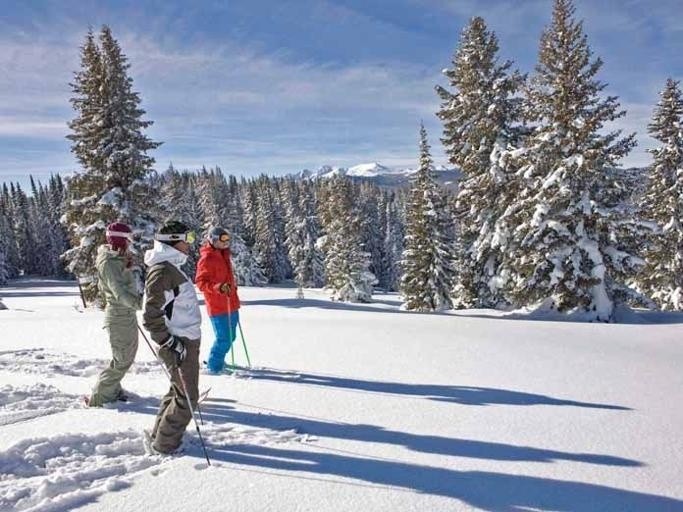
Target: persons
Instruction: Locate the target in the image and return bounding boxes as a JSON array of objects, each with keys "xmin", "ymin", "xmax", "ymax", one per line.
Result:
[
  {"xmin": 88, "ymin": 224, "xmax": 142, "ymax": 406},
  {"xmin": 142, "ymin": 221, "xmax": 202, "ymax": 453},
  {"xmin": 195, "ymin": 227, "xmax": 240, "ymax": 375}
]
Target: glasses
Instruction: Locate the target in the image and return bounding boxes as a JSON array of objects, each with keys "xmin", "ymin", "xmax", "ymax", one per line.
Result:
[
  {"xmin": 105, "ymin": 230, "xmax": 142, "ymax": 245},
  {"xmin": 212, "ymin": 233, "xmax": 230, "ymax": 241},
  {"xmin": 155, "ymin": 230, "xmax": 196, "ymax": 244}
]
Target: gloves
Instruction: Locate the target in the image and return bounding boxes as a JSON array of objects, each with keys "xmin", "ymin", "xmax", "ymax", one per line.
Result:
[
  {"xmin": 221, "ymin": 282, "xmax": 231, "ymax": 295},
  {"xmin": 161, "ymin": 335, "xmax": 188, "ymax": 362}
]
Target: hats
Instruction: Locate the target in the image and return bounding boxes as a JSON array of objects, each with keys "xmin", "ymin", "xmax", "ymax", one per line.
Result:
[
  {"xmin": 158, "ymin": 220, "xmax": 190, "ymax": 246},
  {"xmin": 208, "ymin": 227, "xmax": 226, "ymax": 245},
  {"xmin": 105, "ymin": 222, "xmax": 130, "ymax": 246}
]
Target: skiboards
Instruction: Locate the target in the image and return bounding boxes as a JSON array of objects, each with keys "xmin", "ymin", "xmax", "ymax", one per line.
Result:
[
  {"xmin": 144, "ymin": 422, "xmax": 310, "ymax": 456},
  {"xmin": 83, "ymin": 387, "xmax": 211, "ymax": 407},
  {"xmin": 201, "ymin": 359, "xmax": 302, "ymax": 381}
]
